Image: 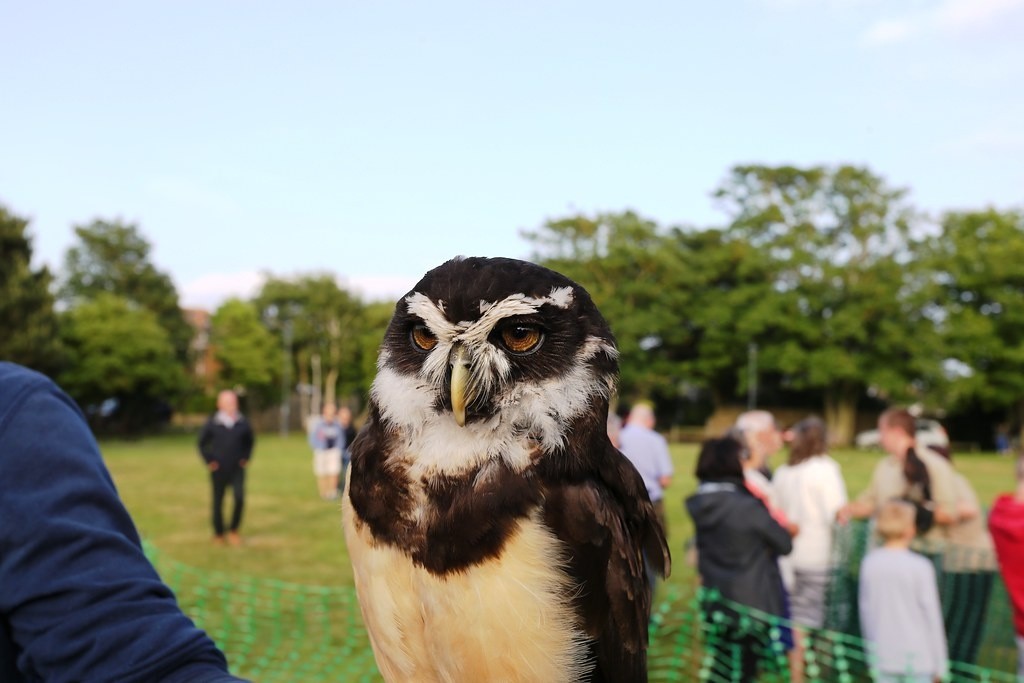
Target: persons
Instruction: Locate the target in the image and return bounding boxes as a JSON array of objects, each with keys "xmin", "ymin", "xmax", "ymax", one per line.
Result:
[
  {"xmin": 305, "ymin": 400, "xmax": 358, "ymax": 502},
  {"xmin": 0, "ymin": 360, "xmax": 251, "ymax": 683},
  {"xmin": 685, "ymin": 406, "xmax": 1024, "ymax": 683},
  {"xmin": 196, "ymin": 386, "xmax": 257, "ymax": 548},
  {"xmin": 618, "ymin": 398, "xmax": 672, "ymax": 542}
]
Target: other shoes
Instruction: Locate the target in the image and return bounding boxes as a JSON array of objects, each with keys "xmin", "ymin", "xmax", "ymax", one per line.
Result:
[{"xmin": 212, "ymin": 532, "xmax": 242, "ymax": 546}]
